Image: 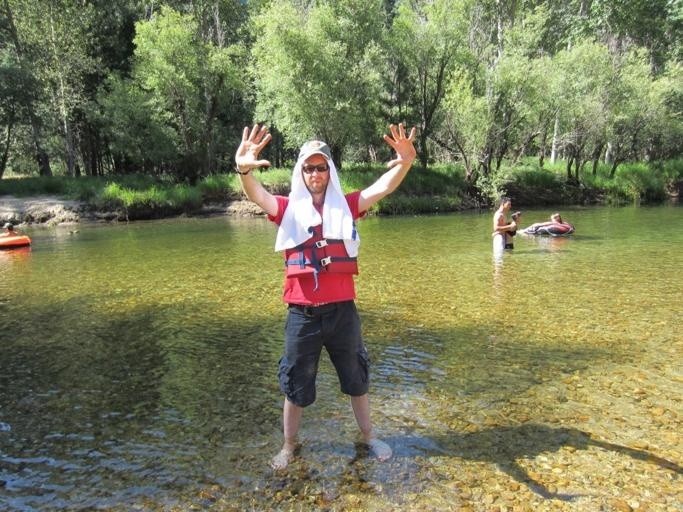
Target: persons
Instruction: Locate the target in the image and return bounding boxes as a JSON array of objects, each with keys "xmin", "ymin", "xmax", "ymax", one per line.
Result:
[
  {"xmin": 0, "ymin": 223, "xmax": 19, "ymax": 238},
  {"xmin": 492, "ymin": 197, "xmax": 514, "ymax": 252},
  {"xmin": 492, "ymin": 212, "xmax": 522, "ymax": 249},
  {"xmin": 551, "ymin": 213, "xmax": 562, "ymax": 224},
  {"xmin": 235, "ymin": 121, "xmax": 417, "ymax": 472}
]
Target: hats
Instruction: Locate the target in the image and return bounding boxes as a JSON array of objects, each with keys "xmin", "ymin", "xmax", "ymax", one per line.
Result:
[
  {"xmin": 2, "ymin": 222, "xmax": 13, "ymax": 228},
  {"xmin": 299, "ymin": 140, "xmax": 331, "ymax": 160}
]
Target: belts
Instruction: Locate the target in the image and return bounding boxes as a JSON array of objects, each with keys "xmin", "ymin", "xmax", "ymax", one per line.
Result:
[{"xmin": 289, "ymin": 299, "xmax": 354, "ymax": 315}]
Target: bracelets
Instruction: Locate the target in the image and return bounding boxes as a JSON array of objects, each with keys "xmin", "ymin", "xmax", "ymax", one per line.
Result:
[{"xmin": 235, "ymin": 168, "xmax": 251, "ymax": 175}]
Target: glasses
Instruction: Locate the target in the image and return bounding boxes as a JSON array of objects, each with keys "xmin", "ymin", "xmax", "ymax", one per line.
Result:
[{"xmin": 303, "ymin": 163, "xmax": 328, "ymax": 174}]
